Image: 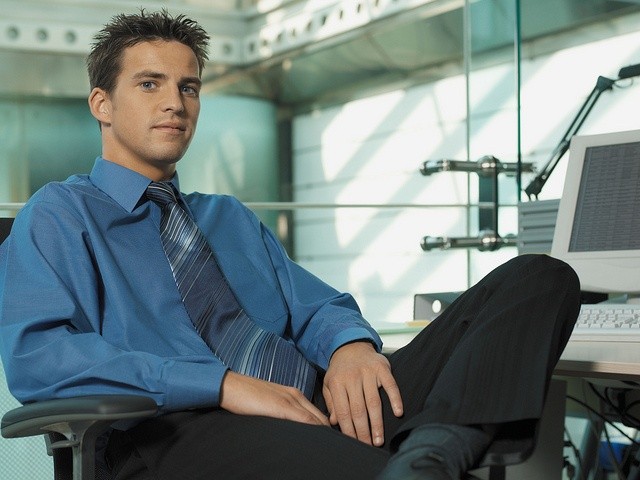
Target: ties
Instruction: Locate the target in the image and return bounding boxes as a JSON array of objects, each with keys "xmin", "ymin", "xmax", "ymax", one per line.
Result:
[{"xmin": 146, "ymin": 181, "xmax": 317, "ymax": 403}]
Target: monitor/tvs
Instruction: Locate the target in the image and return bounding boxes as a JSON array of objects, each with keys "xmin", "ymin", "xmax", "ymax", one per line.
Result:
[{"xmin": 549, "ymin": 130, "xmax": 640, "ymax": 304}]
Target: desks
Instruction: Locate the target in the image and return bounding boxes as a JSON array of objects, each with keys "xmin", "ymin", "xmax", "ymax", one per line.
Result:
[{"xmin": 379, "ymin": 296, "xmax": 640, "ymax": 480}]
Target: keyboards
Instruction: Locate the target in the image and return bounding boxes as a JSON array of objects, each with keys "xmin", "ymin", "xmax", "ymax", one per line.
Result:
[{"xmin": 569, "ymin": 305, "xmax": 640, "ymax": 342}]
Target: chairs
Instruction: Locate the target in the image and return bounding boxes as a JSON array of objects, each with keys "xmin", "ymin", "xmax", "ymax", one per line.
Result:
[{"xmin": 0, "ymin": 217, "xmax": 506, "ymax": 480}]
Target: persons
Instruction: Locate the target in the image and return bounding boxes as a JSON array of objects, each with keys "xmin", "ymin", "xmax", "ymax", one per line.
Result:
[{"xmin": 0, "ymin": 7, "xmax": 580, "ymax": 479}]
